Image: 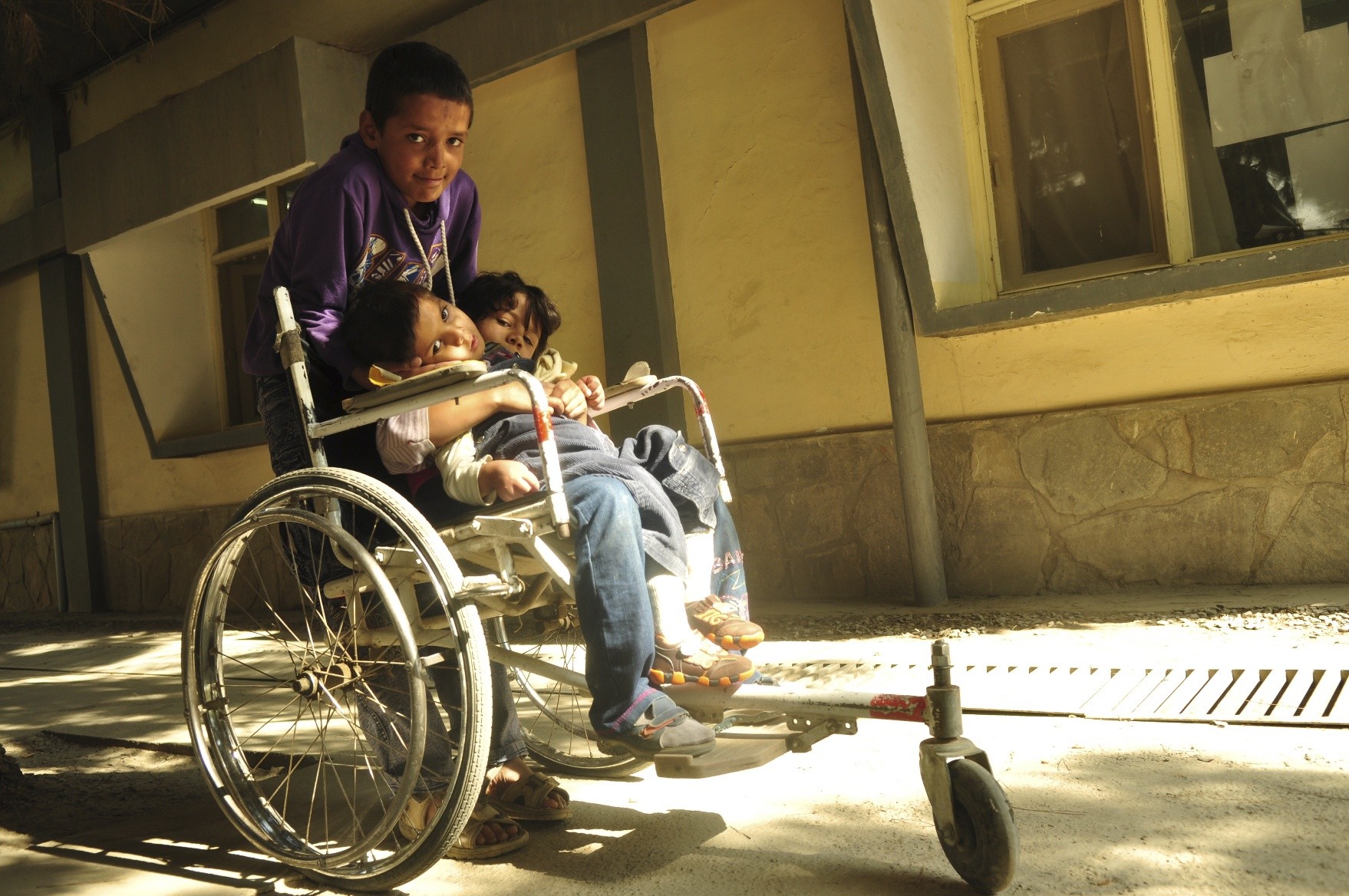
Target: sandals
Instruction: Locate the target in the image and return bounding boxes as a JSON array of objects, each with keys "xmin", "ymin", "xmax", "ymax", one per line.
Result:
[
  {"xmin": 477, "ymin": 756, "xmax": 573, "ymax": 820},
  {"xmin": 399, "ymin": 789, "xmax": 530, "ymax": 859}
]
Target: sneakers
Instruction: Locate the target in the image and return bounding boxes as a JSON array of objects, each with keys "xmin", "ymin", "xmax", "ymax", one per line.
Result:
[
  {"xmin": 685, "ymin": 594, "xmax": 764, "ymax": 649},
  {"xmin": 648, "ymin": 629, "xmax": 754, "ymax": 686},
  {"xmin": 597, "ymin": 697, "xmax": 716, "ymax": 761},
  {"xmin": 699, "ymin": 677, "xmax": 782, "ymax": 732}
]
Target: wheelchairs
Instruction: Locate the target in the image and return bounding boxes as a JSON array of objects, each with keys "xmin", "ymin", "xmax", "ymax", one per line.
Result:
[{"xmin": 179, "ymin": 285, "xmax": 1020, "ymax": 895}]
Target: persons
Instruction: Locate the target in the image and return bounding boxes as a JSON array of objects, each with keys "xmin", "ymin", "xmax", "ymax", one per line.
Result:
[
  {"xmin": 349, "ymin": 270, "xmax": 764, "ymax": 760},
  {"xmin": 247, "ymin": 39, "xmax": 575, "ymax": 858}
]
